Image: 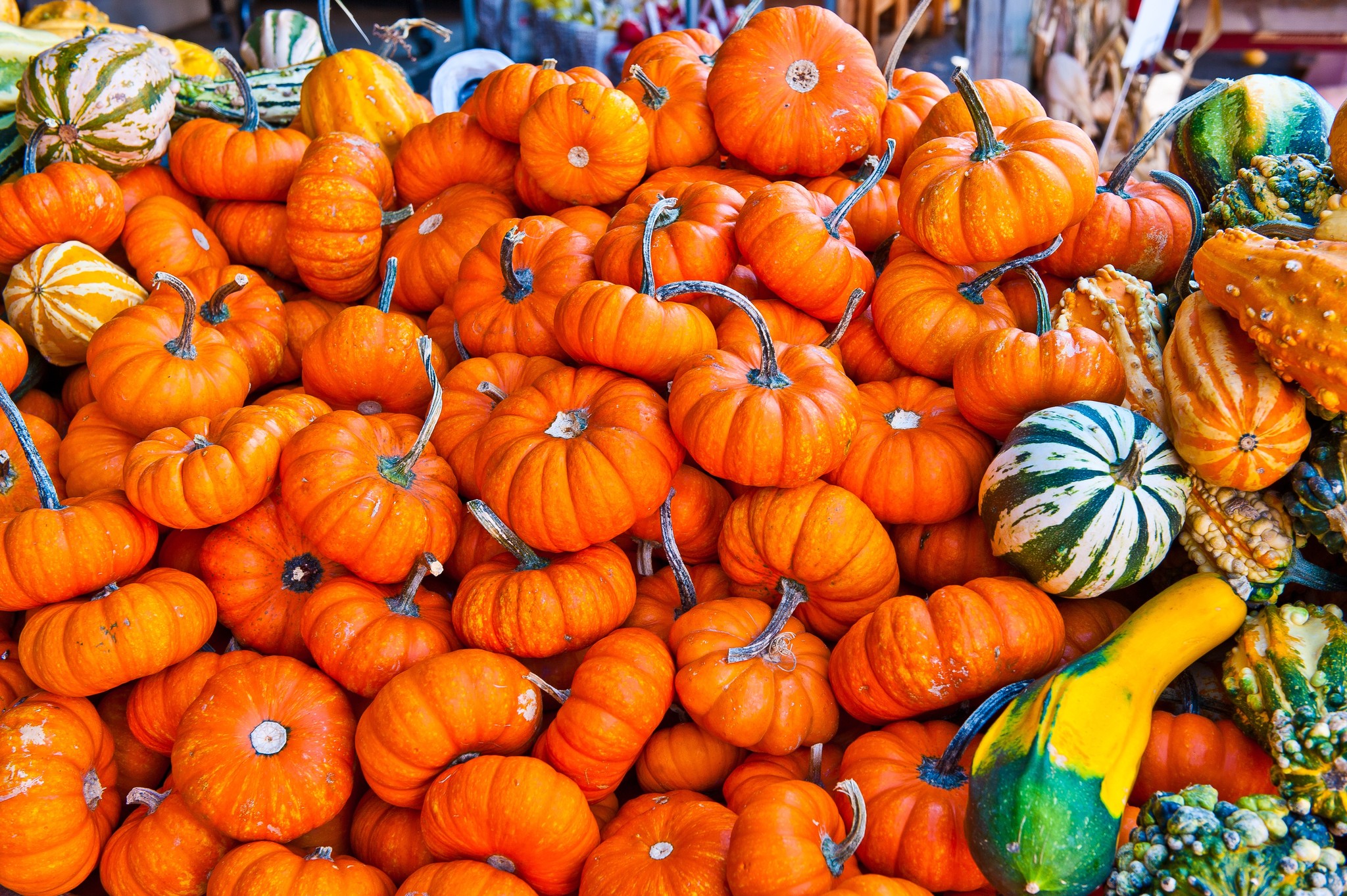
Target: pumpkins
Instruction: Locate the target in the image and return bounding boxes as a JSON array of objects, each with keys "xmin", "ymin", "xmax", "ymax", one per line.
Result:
[{"xmin": 0, "ymin": 0, "xmax": 1346, "ymax": 896}]
